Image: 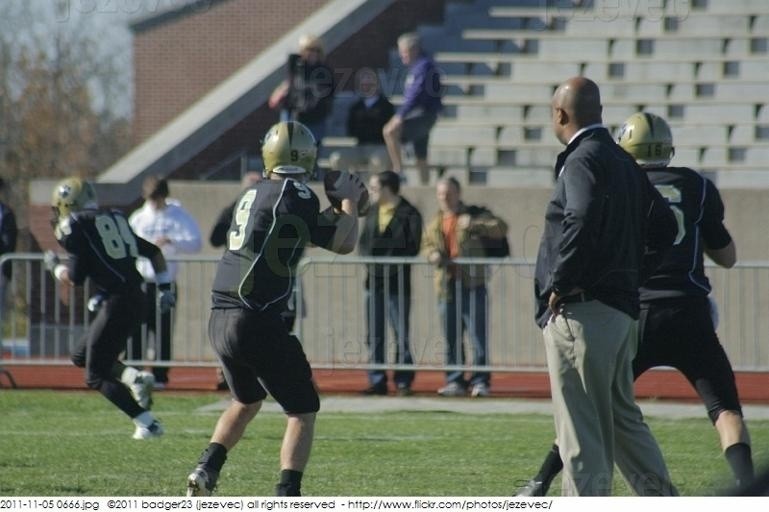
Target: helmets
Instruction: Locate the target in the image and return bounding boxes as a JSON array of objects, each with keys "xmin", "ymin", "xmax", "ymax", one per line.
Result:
[
  {"xmin": 612, "ymin": 112, "xmax": 675, "ymax": 170},
  {"xmin": 48, "ymin": 175, "xmax": 100, "ymax": 230},
  {"xmin": 261, "ymin": 121, "xmax": 321, "ymax": 180}
]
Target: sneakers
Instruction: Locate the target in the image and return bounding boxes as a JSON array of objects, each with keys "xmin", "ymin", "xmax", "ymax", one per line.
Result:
[{"xmin": 130, "ymin": 369, "xmax": 548, "ymax": 495}]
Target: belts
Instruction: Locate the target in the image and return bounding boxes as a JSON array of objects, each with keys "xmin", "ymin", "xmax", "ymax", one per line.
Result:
[{"xmin": 558, "ymin": 292, "xmax": 593, "ymax": 304}]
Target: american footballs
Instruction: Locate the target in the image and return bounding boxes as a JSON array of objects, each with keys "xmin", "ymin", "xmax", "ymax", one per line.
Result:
[{"xmin": 324, "ymin": 170, "xmax": 370, "ymax": 216}]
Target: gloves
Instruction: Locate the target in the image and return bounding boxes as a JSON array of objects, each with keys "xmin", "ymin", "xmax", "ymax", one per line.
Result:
[
  {"xmin": 43, "ymin": 247, "xmax": 60, "ymax": 282},
  {"xmin": 158, "ymin": 289, "xmax": 176, "ymax": 315}
]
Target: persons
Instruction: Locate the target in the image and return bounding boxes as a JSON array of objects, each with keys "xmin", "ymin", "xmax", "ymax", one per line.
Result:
[
  {"xmin": 343, "ymin": 30, "xmax": 441, "ymax": 181},
  {"xmin": 209, "ymin": 169, "xmax": 262, "ymax": 392},
  {"xmin": 359, "ymin": 172, "xmax": 422, "ymax": 396},
  {"xmin": 125, "ymin": 175, "xmax": 202, "ymax": 391},
  {"xmin": 186, "ymin": 119, "xmax": 365, "ymax": 497},
  {"xmin": 0, "ymin": 178, "xmax": 17, "ymax": 341},
  {"xmin": 426, "ymin": 176, "xmax": 508, "ymax": 398},
  {"xmin": 270, "ymin": 36, "xmax": 335, "ymax": 149},
  {"xmin": 533, "ymin": 73, "xmax": 678, "ymax": 497},
  {"xmin": 45, "ymin": 176, "xmax": 175, "ymax": 440},
  {"xmin": 515, "ymin": 111, "xmax": 764, "ymax": 497}
]
